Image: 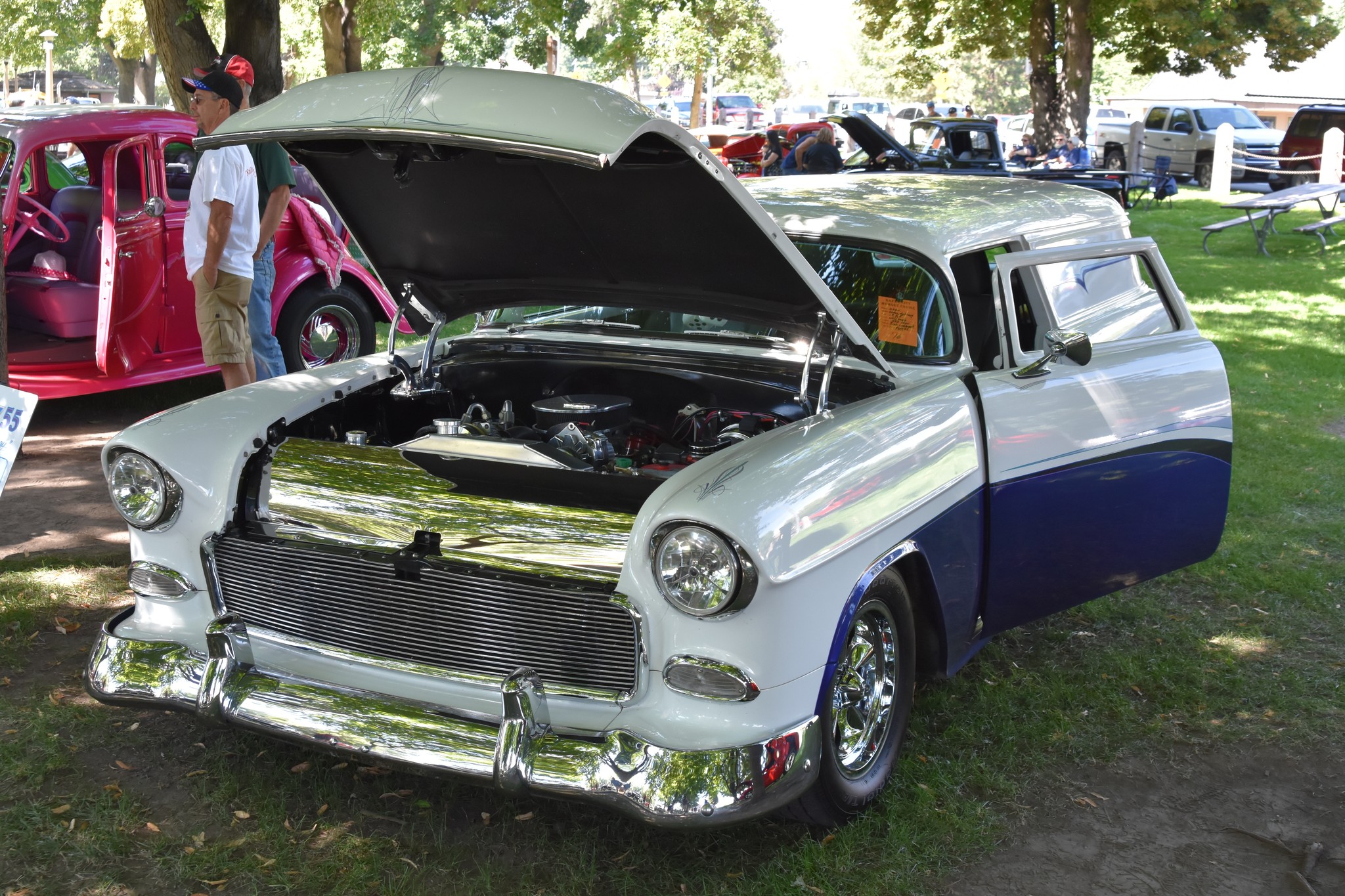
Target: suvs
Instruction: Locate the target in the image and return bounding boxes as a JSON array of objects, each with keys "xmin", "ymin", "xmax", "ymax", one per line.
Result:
[
  {"xmin": 82, "ymin": 62, "xmax": 1238, "ymax": 833},
  {"xmin": 1141, "ymin": 102, "xmax": 1288, "ymax": 192},
  {"xmin": 1276, "ymin": 102, "xmax": 1345, "ymax": 187}
]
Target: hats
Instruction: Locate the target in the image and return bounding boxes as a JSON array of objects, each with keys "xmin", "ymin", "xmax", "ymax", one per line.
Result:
[
  {"xmin": 926, "ymin": 101, "xmax": 934, "ymax": 107},
  {"xmin": 181, "ymin": 71, "xmax": 244, "ymax": 109},
  {"xmin": 947, "ymin": 107, "xmax": 956, "ymax": 114},
  {"xmin": 193, "ymin": 53, "xmax": 254, "ymax": 88}
]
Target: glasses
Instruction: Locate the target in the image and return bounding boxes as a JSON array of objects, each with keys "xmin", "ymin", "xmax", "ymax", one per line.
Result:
[
  {"xmin": 191, "ymin": 94, "xmax": 231, "ymax": 105},
  {"xmin": 1021, "ymin": 139, "xmax": 1030, "ymax": 142},
  {"xmin": 1056, "ymin": 138, "xmax": 1065, "ymax": 141},
  {"xmin": 1067, "ymin": 141, "xmax": 1072, "ymax": 143}
]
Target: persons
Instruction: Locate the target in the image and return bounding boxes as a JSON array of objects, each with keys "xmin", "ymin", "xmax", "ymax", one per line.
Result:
[
  {"xmin": 9, "ymin": 96, "xmax": 81, "ymax": 158},
  {"xmin": 920, "ymin": 100, "xmax": 986, "ymax": 151},
  {"xmin": 177, "ymin": 70, "xmax": 256, "ymax": 391},
  {"xmin": 758, "ymin": 127, "xmax": 846, "ymax": 176},
  {"xmin": 1006, "ymin": 131, "xmax": 1093, "ymax": 172},
  {"xmin": 190, "ymin": 54, "xmax": 298, "ymax": 381}
]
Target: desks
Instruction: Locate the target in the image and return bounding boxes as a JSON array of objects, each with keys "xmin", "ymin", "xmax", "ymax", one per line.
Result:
[{"xmin": 1219, "ymin": 182, "xmax": 1345, "ymax": 260}]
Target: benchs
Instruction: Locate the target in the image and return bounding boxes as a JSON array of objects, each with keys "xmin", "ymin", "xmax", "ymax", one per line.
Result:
[
  {"xmin": 1200, "ymin": 204, "xmax": 1297, "ymax": 255},
  {"xmin": 3, "ymin": 185, "xmax": 191, "ymax": 340},
  {"xmin": 1292, "ymin": 216, "xmax": 1345, "ymax": 256}
]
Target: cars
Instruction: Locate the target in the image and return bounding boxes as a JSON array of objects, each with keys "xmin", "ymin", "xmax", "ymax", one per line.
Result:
[
  {"xmin": 641, "ymin": 87, "xmax": 1141, "ymax": 212},
  {"xmin": 0, "ymin": 101, "xmax": 418, "ymax": 404}
]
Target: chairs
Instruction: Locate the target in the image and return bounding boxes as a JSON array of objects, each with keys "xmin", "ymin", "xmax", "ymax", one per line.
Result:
[
  {"xmin": 897, "ymin": 250, "xmax": 1000, "ymax": 371},
  {"xmin": 974, "ymin": 151, "xmax": 994, "ymax": 160},
  {"xmin": 1126, "ymin": 155, "xmax": 1179, "ymax": 212},
  {"xmin": 959, "ymin": 151, "xmax": 973, "ymax": 160}
]
